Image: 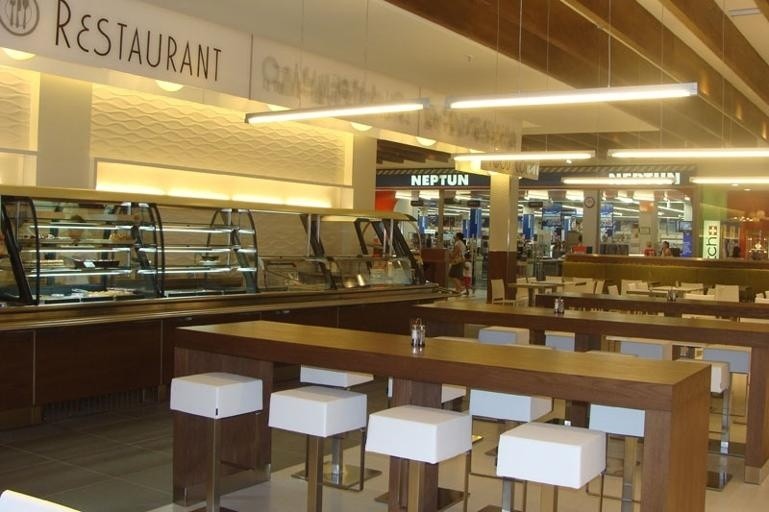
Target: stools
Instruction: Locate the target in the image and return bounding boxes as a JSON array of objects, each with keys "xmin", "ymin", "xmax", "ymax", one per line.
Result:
[
  {"xmin": 362, "ymin": 403, "xmax": 475, "ymax": 511},
  {"xmin": 264, "ymin": 383, "xmax": 369, "ymax": 510},
  {"xmin": 579, "ymin": 400, "xmax": 645, "ymax": 510},
  {"xmin": 492, "ymin": 420, "xmax": 610, "ymax": 511},
  {"xmin": 462, "ymin": 387, "xmax": 564, "ymax": 510},
  {"xmin": 478, "ymin": 324, "xmax": 752, "ymax": 455},
  {"xmin": 292, "ymin": 364, "xmax": 383, "ymax": 509},
  {"xmin": 169, "ymin": 373, "xmax": 265, "ymax": 510}
]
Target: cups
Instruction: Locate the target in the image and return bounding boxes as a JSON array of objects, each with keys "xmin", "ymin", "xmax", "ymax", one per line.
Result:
[
  {"xmin": 667, "ymin": 287, "xmax": 676, "ymax": 301},
  {"xmin": 410, "ymin": 324, "xmax": 427, "ymax": 354},
  {"xmin": 553, "ymin": 298, "xmax": 566, "ymax": 316}
]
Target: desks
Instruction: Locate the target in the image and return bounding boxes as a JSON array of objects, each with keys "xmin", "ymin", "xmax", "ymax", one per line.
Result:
[
  {"xmin": 173, "ymin": 319, "xmax": 711, "ymax": 512},
  {"xmin": 409, "ymin": 298, "xmax": 769, "ymax": 484}
]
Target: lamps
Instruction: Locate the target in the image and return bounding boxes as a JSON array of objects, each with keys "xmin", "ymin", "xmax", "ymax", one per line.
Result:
[
  {"xmin": 244, "ymin": 81, "xmax": 699, "ymax": 126},
  {"xmin": 450, "ymin": 144, "xmax": 769, "ymax": 187}
]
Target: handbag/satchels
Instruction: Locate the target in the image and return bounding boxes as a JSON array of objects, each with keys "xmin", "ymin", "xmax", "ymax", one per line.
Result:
[{"xmin": 450, "ymin": 251, "xmax": 465, "ymax": 265}]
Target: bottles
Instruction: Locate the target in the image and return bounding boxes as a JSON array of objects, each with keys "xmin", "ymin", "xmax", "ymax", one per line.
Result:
[{"xmin": 426, "ymin": 234, "xmax": 431, "ymax": 248}]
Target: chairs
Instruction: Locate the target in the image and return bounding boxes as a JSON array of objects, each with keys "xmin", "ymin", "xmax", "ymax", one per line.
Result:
[{"xmin": 490, "ymin": 275, "xmax": 769, "ymax": 322}]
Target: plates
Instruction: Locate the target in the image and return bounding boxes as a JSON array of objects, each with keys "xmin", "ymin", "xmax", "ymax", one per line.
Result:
[{"xmin": 199, "ymin": 261, "xmax": 220, "ymax": 266}]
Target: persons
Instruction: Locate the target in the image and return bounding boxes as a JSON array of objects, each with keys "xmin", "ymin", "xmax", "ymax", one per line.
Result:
[
  {"xmin": 463, "ymin": 252, "xmax": 476, "ymax": 296},
  {"xmin": 448, "ymin": 233, "xmax": 466, "ymax": 292},
  {"xmin": 660, "ymin": 241, "xmax": 672, "ymax": 257}
]
